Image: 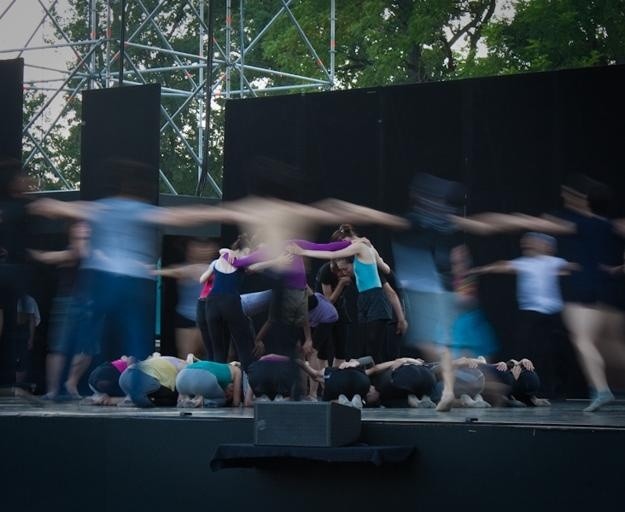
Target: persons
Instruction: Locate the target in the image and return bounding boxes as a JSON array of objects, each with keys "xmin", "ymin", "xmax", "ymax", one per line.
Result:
[{"xmin": 1, "ymin": 160, "xmax": 625, "ymax": 412}]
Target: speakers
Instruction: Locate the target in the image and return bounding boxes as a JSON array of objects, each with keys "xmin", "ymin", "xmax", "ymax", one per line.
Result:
[{"xmin": 253, "ymin": 401, "xmax": 361, "ymax": 448}]
